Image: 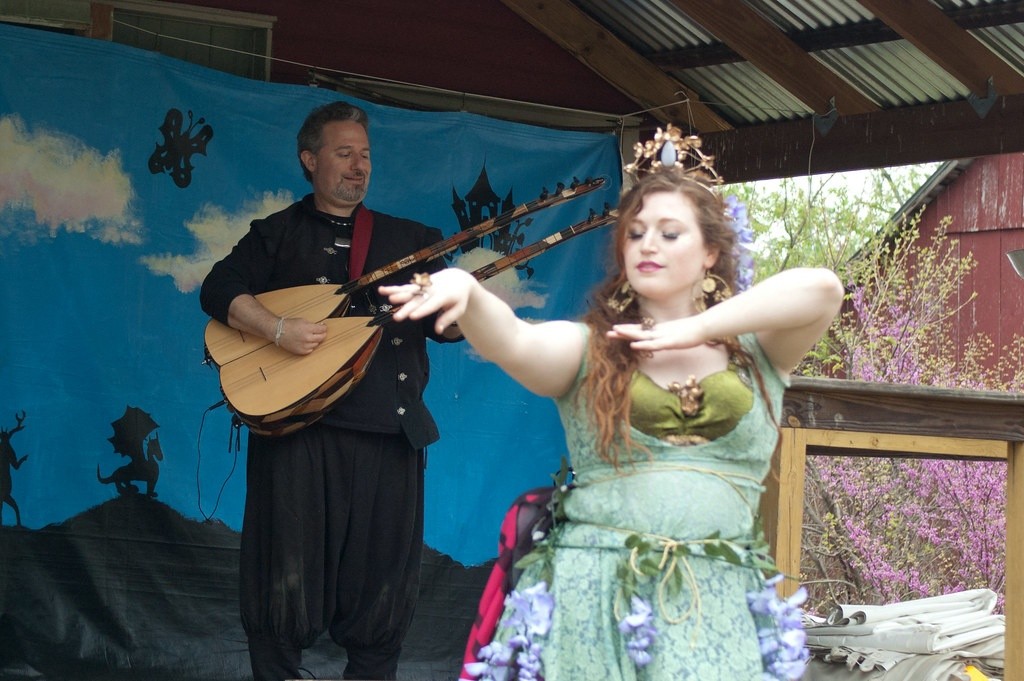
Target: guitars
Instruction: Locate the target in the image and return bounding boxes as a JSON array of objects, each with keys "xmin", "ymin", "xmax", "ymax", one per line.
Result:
[{"xmin": 202, "ymin": 175, "xmax": 624, "ymax": 436}]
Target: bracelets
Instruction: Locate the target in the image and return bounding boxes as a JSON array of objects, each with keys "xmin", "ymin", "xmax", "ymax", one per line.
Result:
[
  {"xmin": 276, "ymin": 316, "xmax": 284, "ymax": 346},
  {"xmin": 451, "ymin": 324, "xmax": 458, "ymax": 327}
]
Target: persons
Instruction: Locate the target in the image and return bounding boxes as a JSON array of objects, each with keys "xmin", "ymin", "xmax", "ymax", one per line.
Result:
[
  {"xmin": 379, "ymin": 128, "xmax": 843, "ymax": 681},
  {"xmin": 200, "ymin": 101, "xmax": 466, "ymax": 681}
]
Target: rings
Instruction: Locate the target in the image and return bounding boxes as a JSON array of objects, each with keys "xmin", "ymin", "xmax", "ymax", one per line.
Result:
[
  {"xmin": 411, "ymin": 272, "xmax": 431, "ymax": 299},
  {"xmin": 642, "ymin": 317, "xmax": 655, "ymax": 330}
]
